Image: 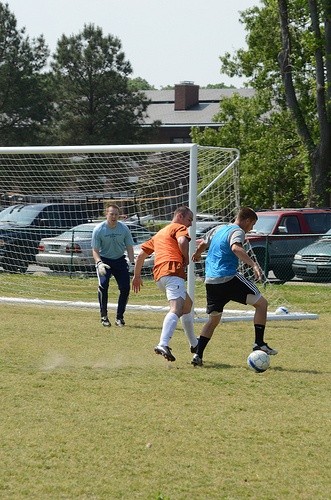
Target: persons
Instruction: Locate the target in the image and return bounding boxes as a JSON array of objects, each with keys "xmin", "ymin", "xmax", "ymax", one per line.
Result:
[
  {"xmin": 132, "ymin": 206, "xmax": 200, "ymax": 362},
  {"xmin": 91, "ymin": 202, "xmax": 136, "ymax": 328},
  {"xmin": 190, "ymin": 207, "xmax": 278, "ymax": 367}
]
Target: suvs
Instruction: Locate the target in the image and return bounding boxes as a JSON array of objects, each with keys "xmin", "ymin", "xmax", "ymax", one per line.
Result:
[{"xmin": 197, "ymin": 206, "xmax": 331, "ymax": 283}]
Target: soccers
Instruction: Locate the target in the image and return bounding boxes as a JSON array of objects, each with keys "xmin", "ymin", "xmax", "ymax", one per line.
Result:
[
  {"xmin": 275, "ymin": 307, "xmax": 289, "ymax": 315},
  {"xmin": 247, "ymin": 350, "xmax": 271, "ymax": 373}
]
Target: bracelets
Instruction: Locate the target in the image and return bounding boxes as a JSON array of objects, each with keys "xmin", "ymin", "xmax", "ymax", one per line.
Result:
[{"xmin": 96, "ymin": 260, "xmax": 102, "ymax": 264}]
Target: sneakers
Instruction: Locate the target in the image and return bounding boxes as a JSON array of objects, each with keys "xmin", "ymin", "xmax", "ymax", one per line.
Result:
[
  {"xmin": 115, "ymin": 317, "xmax": 125, "ymax": 327},
  {"xmin": 101, "ymin": 316, "xmax": 111, "ymax": 327},
  {"xmin": 191, "ymin": 335, "xmax": 201, "ymax": 353},
  {"xmin": 191, "ymin": 354, "xmax": 204, "ymax": 366},
  {"xmin": 253, "ymin": 343, "xmax": 278, "ymax": 355},
  {"xmin": 155, "ymin": 345, "xmax": 176, "ymax": 361}
]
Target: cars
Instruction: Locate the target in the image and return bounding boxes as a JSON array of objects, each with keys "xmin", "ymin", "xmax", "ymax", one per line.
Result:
[
  {"xmin": 35, "ymin": 222, "xmax": 155, "ymax": 275},
  {"xmin": 126, "ymin": 221, "xmax": 230, "ymax": 279},
  {"xmin": 291, "ymin": 228, "xmax": 331, "ymax": 280},
  {"xmin": 0, "ymin": 203, "xmax": 100, "ymax": 272}
]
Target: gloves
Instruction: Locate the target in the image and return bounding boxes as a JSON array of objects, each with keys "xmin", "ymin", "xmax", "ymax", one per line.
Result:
[
  {"xmin": 128, "ymin": 262, "xmax": 135, "ymax": 273},
  {"xmin": 97, "ymin": 262, "xmax": 110, "ymax": 277}
]
